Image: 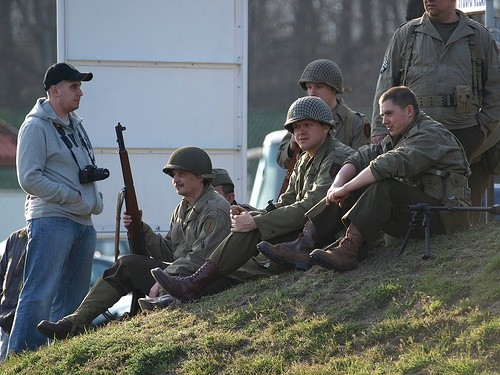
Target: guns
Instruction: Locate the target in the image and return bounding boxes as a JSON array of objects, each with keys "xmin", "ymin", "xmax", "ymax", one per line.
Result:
[{"xmin": 275, "ymin": 144, "xmax": 302, "ymax": 202}]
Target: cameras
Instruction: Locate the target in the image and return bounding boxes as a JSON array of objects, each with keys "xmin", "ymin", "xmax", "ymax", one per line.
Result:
[{"xmin": 80, "ymin": 165, "xmax": 110, "ymax": 184}]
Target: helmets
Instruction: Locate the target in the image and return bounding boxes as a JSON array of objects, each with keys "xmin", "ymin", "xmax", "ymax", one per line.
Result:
[
  {"xmin": 162, "ymin": 146, "xmax": 212, "ymax": 182},
  {"xmin": 298, "ymin": 60, "xmax": 343, "ymax": 94},
  {"xmin": 284, "ymin": 96, "xmax": 336, "ymax": 135}
]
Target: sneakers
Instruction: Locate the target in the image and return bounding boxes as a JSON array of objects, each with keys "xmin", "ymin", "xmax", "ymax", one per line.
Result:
[
  {"xmin": 256, "ymin": 227, "xmax": 318, "ymax": 271},
  {"xmin": 36, "ymin": 317, "xmax": 86, "ymax": 340},
  {"xmin": 309, "ymin": 233, "xmax": 360, "ymax": 271}
]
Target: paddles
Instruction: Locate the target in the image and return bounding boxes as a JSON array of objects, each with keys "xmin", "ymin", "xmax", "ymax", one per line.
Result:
[{"xmin": 114, "ymin": 122, "xmax": 154, "ymax": 318}]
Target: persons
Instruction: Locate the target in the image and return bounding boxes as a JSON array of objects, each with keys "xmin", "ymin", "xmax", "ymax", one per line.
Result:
[
  {"xmin": 4, "ymin": 62, "xmax": 105, "ymax": 359},
  {"xmin": 257, "ymin": 88, "xmax": 472, "ymax": 272},
  {"xmin": 36, "ymin": 147, "xmax": 234, "ymax": 343},
  {"xmin": 210, "ymin": 168, "xmax": 259, "ymax": 212},
  {"xmin": 150, "ymin": 96, "xmax": 357, "ymax": 304},
  {"xmin": 275, "ymin": 60, "xmax": 372, "ymax": 169},
  {"xmin": 371, "ymin": 0, "xmax": 500, "ymax": 227},
  {"xmin": 0, "ymin": 220, "xmax": 29, "ymax": 360}
]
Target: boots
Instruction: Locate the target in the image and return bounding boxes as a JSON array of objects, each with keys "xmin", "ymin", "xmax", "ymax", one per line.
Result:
[
  {"xmin": 149, "ymin": 260, "xmax": 223, "ymax": 303},
  {"xmin": 138, "ymin": 295, "xmax": 175, "ymax": 311}
]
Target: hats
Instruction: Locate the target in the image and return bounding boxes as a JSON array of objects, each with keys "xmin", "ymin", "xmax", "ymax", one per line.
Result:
[
  {"xmin": 43, "ymin": 63, "xmax": 93, "ymax": 92},
  {"xmin": 210, "ymin": 169, "xmax": 234, "ymax": 187}
]
